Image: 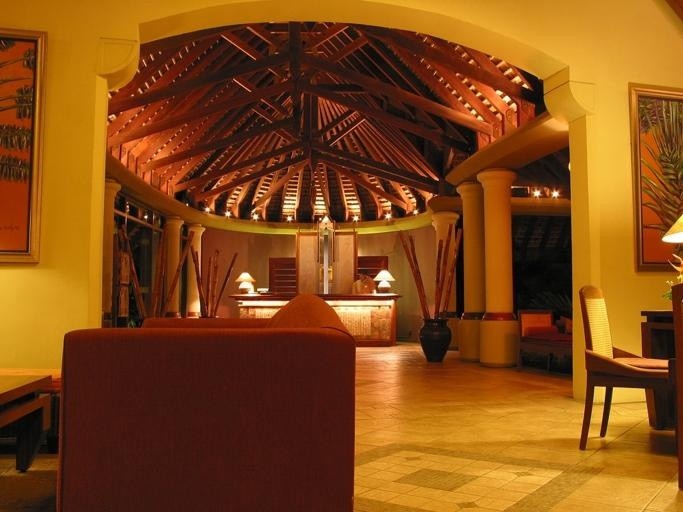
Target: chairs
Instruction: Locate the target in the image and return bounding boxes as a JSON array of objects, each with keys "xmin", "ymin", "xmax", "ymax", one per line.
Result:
[
  {"xmin": 518, "ymin": 310, "xmax": 551, "ymax": 373},
  {"xmin": 579, "ymin": 284, "xmax": 680, "ymax": 450}
]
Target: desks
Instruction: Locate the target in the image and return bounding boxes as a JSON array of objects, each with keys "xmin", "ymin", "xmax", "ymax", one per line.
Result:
[
  {"xmin": 229, "ymin": 294, "xmax": 403, "ymax": 347},
  {"xmin": 0, "ymin": 375, "xmax": 53, "ymax": 474},
  {"xmin": 519, "ymin": 335, "xmax": 572, "ymax": 354}
]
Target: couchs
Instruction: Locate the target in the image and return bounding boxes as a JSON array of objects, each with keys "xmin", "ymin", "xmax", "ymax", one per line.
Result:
[{"xmin": 54, "ymin": 291, "xmax": 356, "ymax": 512}]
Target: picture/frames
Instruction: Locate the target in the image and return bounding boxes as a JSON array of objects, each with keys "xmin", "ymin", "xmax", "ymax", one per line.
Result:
[
  {"xmin": 0, "ymin": 28, "xmax": 47, "ymax": 267},
  {"xmin": 628, "ymin": 82, "xmax": 683, "ymax": 273}
]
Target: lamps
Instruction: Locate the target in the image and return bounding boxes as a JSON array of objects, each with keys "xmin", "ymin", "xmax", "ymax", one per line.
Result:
[
  {"xmin": 235, "ymin": 272, "xmax": 256, "ymax": 293},
  {"xmin": 373, "ymin": 269, "xmax": 395, "ymax": 292},
  {"xmin": 660, "ymin": 211, "xmax": 683, "ymax": 247}
]
